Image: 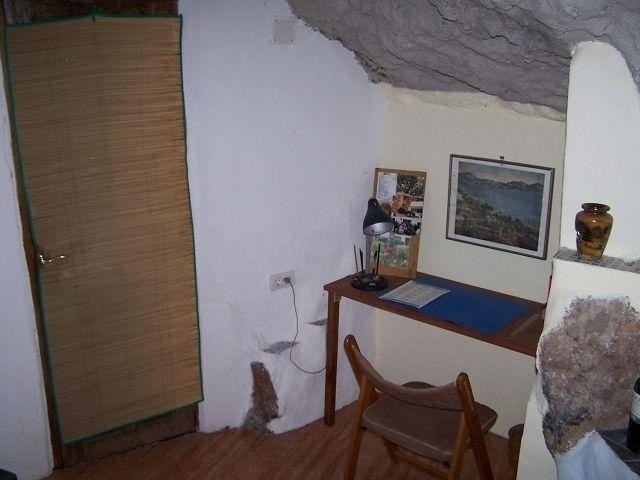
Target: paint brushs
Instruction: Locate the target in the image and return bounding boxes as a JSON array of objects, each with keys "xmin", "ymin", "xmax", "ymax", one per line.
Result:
[{"xmin": 354, "ymin": 243, "xmax": 381, "ymax": 278}]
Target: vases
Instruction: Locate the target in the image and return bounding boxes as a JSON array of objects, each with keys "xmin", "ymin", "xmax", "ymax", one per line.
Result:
[{"xmin": 574, "ymin": 202, "xmax": 613, "ymax": 260}]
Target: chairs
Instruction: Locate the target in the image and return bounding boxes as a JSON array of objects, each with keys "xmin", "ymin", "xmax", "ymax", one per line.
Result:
[{"xmin": 341, "ymin": 333, "xmax": 499, "ymax": 479}]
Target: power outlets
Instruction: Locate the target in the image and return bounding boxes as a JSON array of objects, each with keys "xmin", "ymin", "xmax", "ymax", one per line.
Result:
[{"xmin": 268, "ymin": 269, "xmax": 295, "ymax": 291}]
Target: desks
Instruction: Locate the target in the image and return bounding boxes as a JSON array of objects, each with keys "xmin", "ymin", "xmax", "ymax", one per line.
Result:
[{"xmin": 324, "ymin": 267, "xmax": 543, "ymax": 427}]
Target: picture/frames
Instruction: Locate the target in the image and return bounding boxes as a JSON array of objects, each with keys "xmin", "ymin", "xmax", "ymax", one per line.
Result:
[{"xmin": 445, "ymin": 154, "xmax": 555, "ymax": 263}]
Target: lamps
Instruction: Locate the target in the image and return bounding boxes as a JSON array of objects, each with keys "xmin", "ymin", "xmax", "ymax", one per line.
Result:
[{"xmin": 350, "ymin": 197, "xmax": 396, "ymax": 292}]
